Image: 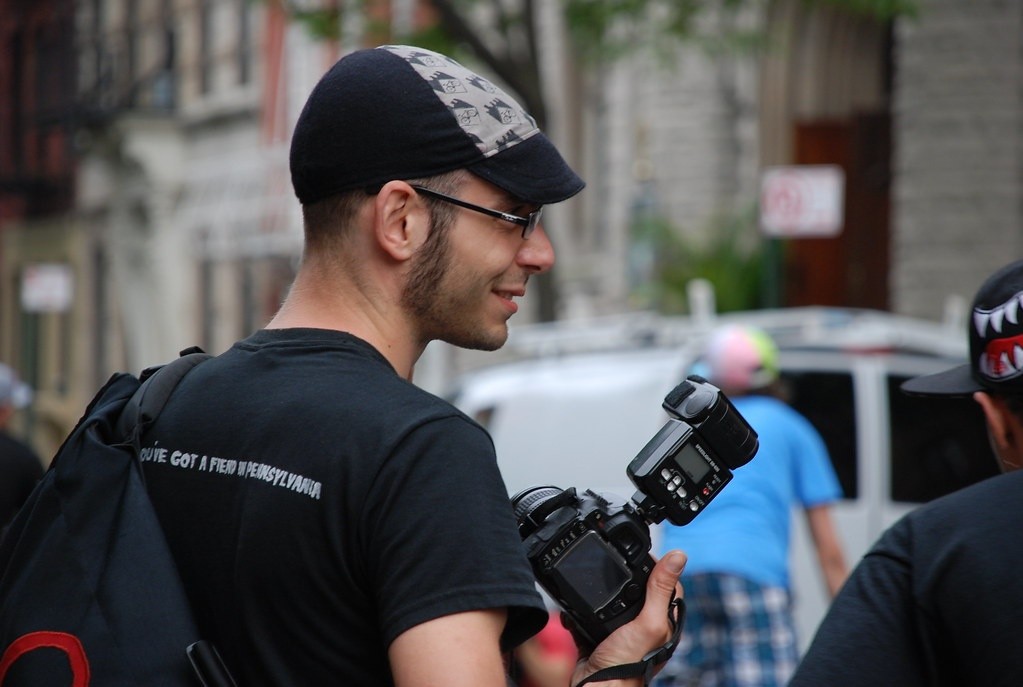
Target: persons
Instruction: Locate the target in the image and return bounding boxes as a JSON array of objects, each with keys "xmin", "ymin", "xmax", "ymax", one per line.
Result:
[
  {"xmin": 649, "ymin": 319, "xmax": 852, "ymax": 687},
  {"xmin": 131, "ymin": 45, "xmax": 687, "ymax": 687},
  {"xmin": 784, "ymin": 261, "xmax": 1022, "ymax": 687},
  {"xmin": 0, "ymin": 361, "xmax": 45, "ymax": 534}
]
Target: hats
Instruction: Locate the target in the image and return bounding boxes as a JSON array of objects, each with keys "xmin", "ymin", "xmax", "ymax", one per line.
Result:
[
  {"xmin": 288, "ymin": 44, "xmax": 585, "ymax": 205},
  {"xmin": 709, "ymin": 326, "xmax": 780, "ymax": 394},
  {"xmin": 901, "ymin": 260, "xmax": 1023, "ymax": 393}
]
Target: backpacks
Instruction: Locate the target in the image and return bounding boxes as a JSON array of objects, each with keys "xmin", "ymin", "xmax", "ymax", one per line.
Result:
[{"xmin": 0, "ymin": 346, "xmax": 231, "ymax": 687}]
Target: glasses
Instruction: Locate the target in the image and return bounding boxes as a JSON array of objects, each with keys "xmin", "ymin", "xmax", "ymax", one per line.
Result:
[{"xmin": 365, "ymin": 182, "xmax": 544, "ymax": 239}]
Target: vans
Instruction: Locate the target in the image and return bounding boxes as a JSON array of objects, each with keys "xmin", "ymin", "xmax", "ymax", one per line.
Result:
[{"xmin": 451, "ymin": 306, "xmax": 999, "ymax": 664}]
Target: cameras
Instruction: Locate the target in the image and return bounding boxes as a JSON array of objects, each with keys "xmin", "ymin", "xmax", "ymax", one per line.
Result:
[{"xmin": 510, "ymin": 375, "xmax": 760, "ymax": 652}]
